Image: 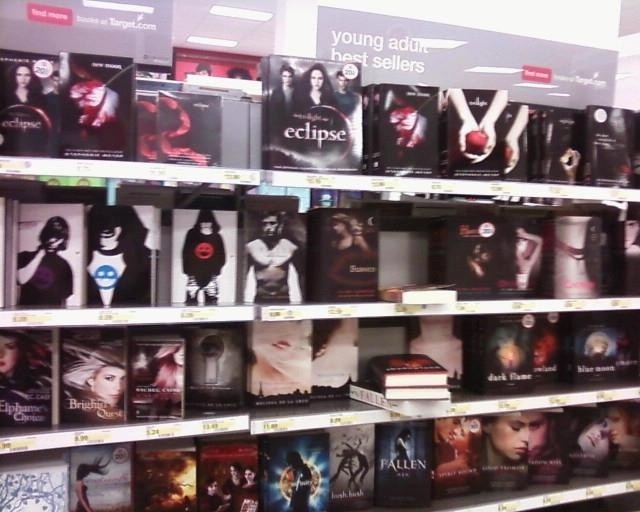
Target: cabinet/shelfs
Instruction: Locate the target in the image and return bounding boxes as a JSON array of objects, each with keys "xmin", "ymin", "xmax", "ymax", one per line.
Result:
[{"xmin": 1, "ymin": 155, "xmax": 640, "ymax": 511}]
